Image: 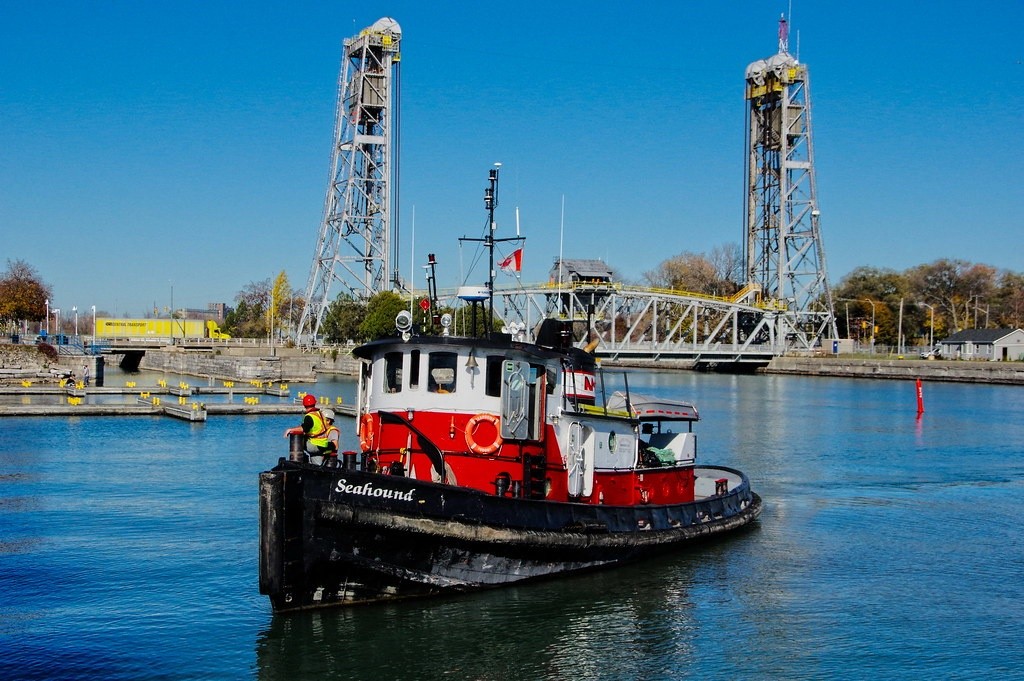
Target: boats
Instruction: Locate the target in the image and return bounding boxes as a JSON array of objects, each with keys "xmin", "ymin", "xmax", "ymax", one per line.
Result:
[{"xmin": 253, "ymin": 163, "xmax": 765, "ymax": 618}]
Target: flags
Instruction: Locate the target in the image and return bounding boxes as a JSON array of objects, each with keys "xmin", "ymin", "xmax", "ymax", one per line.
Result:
[{"xmin": 495, "ymin": 246, "xmax": 523, "ymax": 272}]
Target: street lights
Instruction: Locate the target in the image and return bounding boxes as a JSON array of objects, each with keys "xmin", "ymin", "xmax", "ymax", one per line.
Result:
[
  {"xmin": 51, "ymin": 310, "xmax": 59, "ymax": 336},
  {"xmin": 72, "ymin": 306, "xmax": 78, "ymax": 337},
  {"xmin": 44, "ymin": 298, "xmax": 49, "ymax": 336},
  {"xmin": 91, "ymin": 306, "xmax": 96, "ymax": 345}
]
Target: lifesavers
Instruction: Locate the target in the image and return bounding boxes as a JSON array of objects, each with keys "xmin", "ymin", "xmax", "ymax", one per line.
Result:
[
  {"xmin": 359, "ymin": 414, "xmax": 372, "ymax": 451},
  {"xmin": 464, "ymin": 413, "xmax": 504, "ymax": 456}
]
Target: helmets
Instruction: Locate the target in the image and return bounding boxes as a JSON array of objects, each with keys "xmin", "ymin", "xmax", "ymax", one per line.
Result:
[
  {"xmin": 302, "ymin": 394, "xmax": 317, "ymax": 406},
  {"xmin": 322, "ymin": 408, "xmax": 335, "ymax": 420}
]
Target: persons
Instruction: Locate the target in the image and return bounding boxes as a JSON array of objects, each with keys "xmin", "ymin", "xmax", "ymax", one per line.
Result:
[
  {"xmin": 320, "ymin": 408, "xmax": 340, "ymax": 467},
  {"xmin": 283, "ymin": 393, "xmax": 328, "ymax": 467},
  {"xmin": 82, "ymin": 364, "xmax": 91, "ymax": 387}
]
